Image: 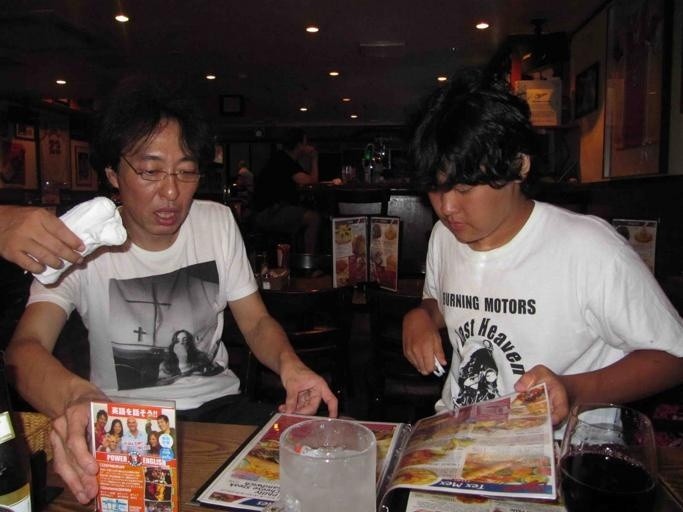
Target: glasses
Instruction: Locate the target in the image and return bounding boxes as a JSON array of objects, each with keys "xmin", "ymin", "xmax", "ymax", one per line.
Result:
[{"xmin": 117, "ymin": 153, "xmax": 205, "ymax": 181}]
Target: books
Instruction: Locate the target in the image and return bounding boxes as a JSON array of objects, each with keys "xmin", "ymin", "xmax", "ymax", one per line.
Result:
[
  {"xmin": 193, "ymin": 381, "xmax": 567, "ymax": 511},
  {"xmin": 328, "ymin": 211, "xmax": 403, "ymax": 295}
]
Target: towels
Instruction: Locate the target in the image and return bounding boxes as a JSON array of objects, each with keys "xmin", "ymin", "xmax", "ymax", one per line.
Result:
[{"xmin": 26, "ymin": 195, "xmax": 127, "ymax": 287}]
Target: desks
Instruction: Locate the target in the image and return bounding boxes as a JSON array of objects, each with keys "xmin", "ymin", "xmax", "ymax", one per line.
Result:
[{"xmin": 0, "ymin": 410, "xmax": 683, "ymax": 512}]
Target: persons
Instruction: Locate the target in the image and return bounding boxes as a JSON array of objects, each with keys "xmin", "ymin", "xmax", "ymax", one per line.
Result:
[
  {"xmin": 248, "ymin": 122, "xmax": 321, "ymax": 254},
  {"xmin": 398, "ymin": 70, "xmax": 682, "ymax": 451},
  {"xmin": 0, "ymin": 72, "xmax": 341, "ymax": 507},
  {"xmin": 234, "ymin": 158, "xmax": 254, "ymax": 193},
  {"xmin": 93, "ymin": 409, "xmax": 176, "ymax": 462},
  {"xmin": 0, "ymin": 202, "xmax": 87, "ymax": 278},
  {"xmin": 156, "ymin": 329, "xmax": 214, "ymax": 382}
]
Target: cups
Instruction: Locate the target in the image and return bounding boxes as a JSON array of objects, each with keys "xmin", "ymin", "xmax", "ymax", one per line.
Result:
[
  {"xmin": 608, "ymin": 47, "xmax": 649, "ymax": 150},
  {"xmin": 279, "ymin": 418, "xmax": 376, "ymax": 512},
  {"xmin": 557, "ymin": 401, "xmax": 661, "ymax": 511}
]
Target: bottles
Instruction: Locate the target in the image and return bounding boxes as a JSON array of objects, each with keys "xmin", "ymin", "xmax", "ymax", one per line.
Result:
[
  {"xmin": 254, "ymin": 273, "xmax": 272, "ymax": 291},
  {"xmin": 0, "ymin": 351, "xmax": 34, "ymax": 512}
]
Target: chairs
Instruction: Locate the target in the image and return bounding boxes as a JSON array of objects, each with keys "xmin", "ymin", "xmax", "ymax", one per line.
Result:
[{"xmin": 231, "ymin": 189, "xmax": 436, "ymax": 398}]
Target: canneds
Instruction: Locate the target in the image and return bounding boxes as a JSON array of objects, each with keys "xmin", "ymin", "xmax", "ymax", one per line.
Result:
[{"xmin": 276, "ymin": 243, "xmax": 291, "ymax": 268}]
